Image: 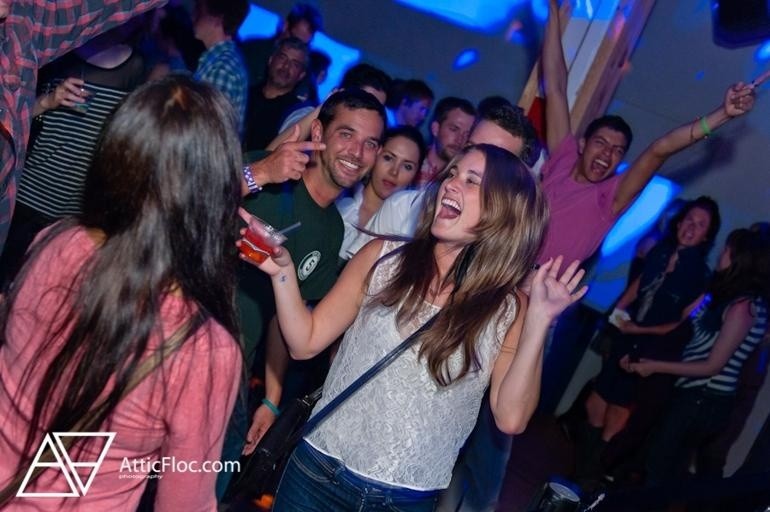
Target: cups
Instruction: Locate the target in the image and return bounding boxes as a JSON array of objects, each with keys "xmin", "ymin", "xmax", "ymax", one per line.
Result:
[
  {"xmin": 240, "ymin": 213, "xmax": 289, "ymax": 264},
  {"xmin": 71, "ymin": 85, "xmax": 97, "ymax": 115}
]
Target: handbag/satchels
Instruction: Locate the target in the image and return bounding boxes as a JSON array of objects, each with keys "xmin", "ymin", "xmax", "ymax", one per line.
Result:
[{"xmin": 228, "ymin": 384, "xmax": 324, "ymax": 503}]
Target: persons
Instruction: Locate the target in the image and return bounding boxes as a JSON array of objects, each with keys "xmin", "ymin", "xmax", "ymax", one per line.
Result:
[{"xmin": 1, "ymin": 0, "xmax": 769, "ymax": 512}]
